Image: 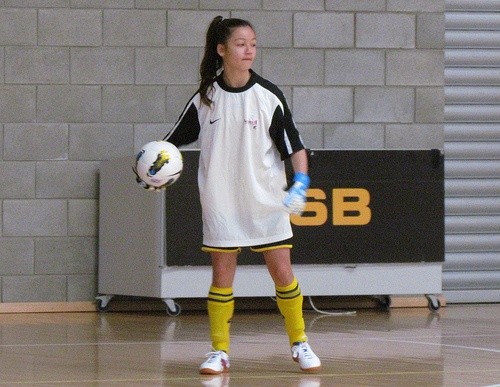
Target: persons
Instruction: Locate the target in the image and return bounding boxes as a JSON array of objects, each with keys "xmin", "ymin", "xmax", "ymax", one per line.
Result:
[{"xmin": 133, "ymin": 16, "xmax": 321, "ymax": 375}]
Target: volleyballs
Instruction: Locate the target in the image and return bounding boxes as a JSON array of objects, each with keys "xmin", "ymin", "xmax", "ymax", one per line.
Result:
[{"xmin": 134, "ymin": 138, "xmax": 184, "ymax": 190}]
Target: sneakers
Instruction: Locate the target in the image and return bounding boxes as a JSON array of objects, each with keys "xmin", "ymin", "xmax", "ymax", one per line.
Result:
[
  {"xmin": 199, "ymin": 351, "xmax": 230, "ymax": 374},
  {"xmin": 292, "ymin": 342, "xmax": 321, "ymax": 371}
]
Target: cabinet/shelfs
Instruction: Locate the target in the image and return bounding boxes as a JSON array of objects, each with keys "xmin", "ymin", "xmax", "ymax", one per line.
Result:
[{"xmin": 96, "ymin": 148, "xmax": 445, "ymax": 316}]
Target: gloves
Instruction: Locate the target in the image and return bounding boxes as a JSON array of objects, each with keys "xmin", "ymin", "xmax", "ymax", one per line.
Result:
[
  {"xmin": 282, "ymin": 173, "xmax": 312, "ymax": 216},
  {"xmin": 136, "ymin": 176, "xmax": 166, "ymax": 193}
]
